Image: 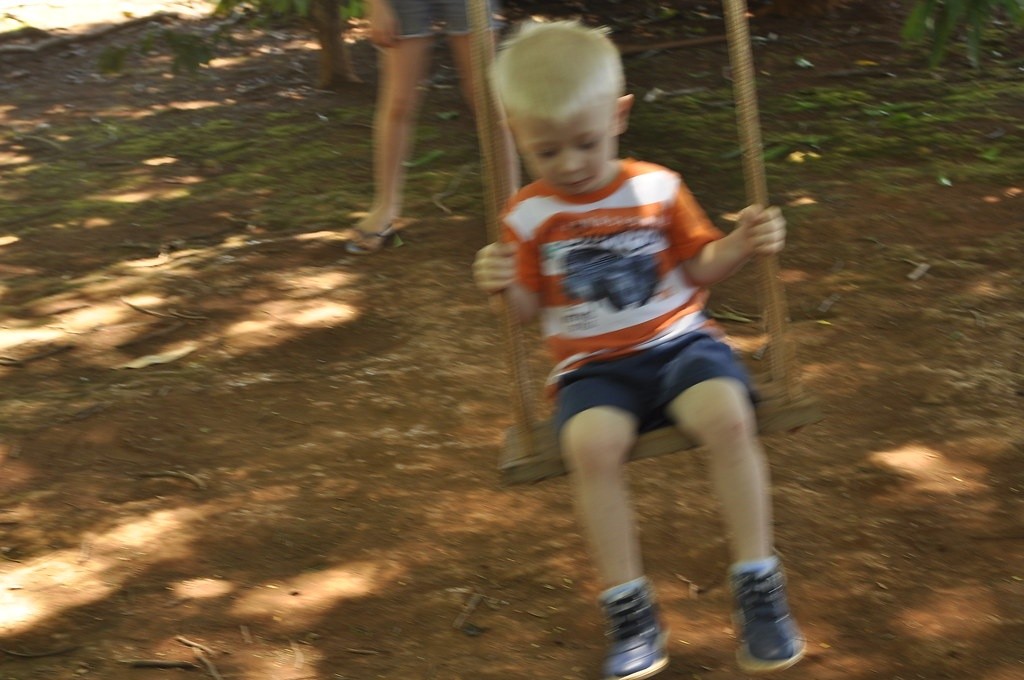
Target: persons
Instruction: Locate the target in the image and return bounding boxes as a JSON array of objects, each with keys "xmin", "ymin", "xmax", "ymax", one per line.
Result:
[
  {"xmin": 345, "ymin": 0, "xmax": 525, "ymax": 256},
  {"xmin": 473, "ymin": 19, "xmax": 806, "ymax": 680}
]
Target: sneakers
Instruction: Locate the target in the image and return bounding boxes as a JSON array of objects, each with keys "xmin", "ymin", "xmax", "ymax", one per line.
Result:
[
  {"xmin": 597, "ymin": 576, "xmax": 669, "ymax": 679},
  {"xmin": 725, "ymin": 558, "xmax": 805, "ymax": 672}
]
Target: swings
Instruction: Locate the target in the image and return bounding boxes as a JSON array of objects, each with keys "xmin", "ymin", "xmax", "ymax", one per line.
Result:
[{"xmin": 470, "ymin": 0, "xmax": 824, "ymax": 489}]
